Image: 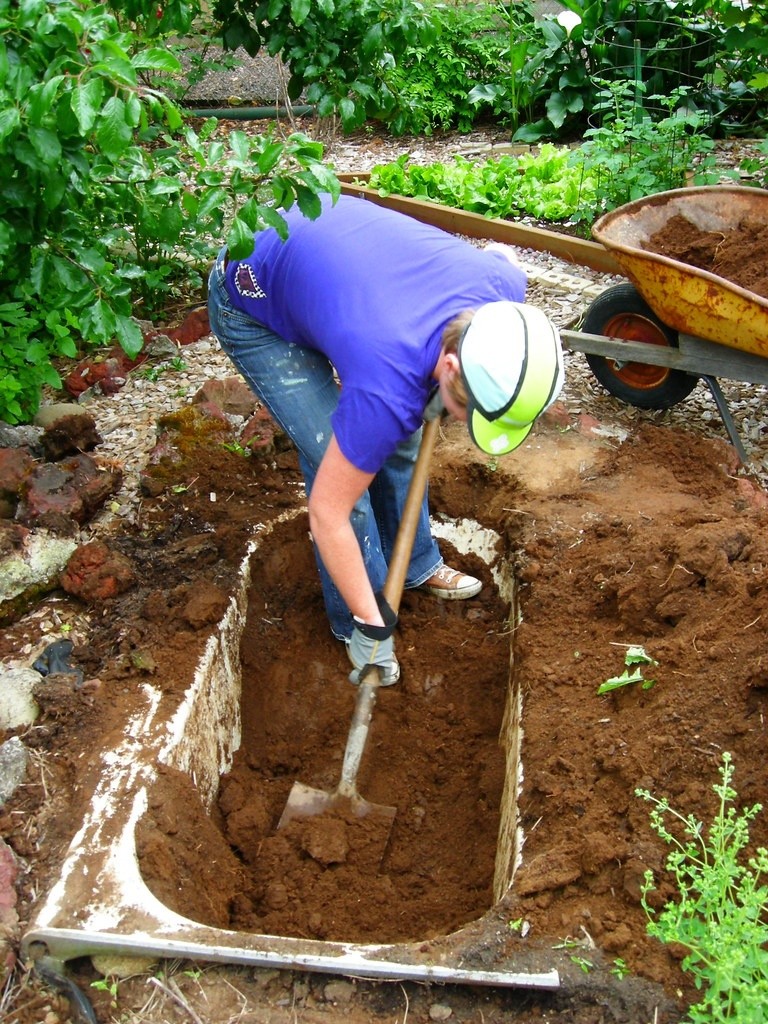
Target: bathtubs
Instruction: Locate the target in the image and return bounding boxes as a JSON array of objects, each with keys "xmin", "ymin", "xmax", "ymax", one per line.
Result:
[{"xmin": 27, "ymin": 506, "xmax": 568, "ymax": 991}]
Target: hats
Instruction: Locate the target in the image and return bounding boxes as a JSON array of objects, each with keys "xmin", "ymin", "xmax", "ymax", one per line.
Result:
[{"xmin": 460, "ymin": 300, "xmax": 566, "ymax": 457}]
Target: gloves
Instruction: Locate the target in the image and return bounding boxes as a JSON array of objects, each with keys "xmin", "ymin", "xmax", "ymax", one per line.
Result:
[
  {"xmin": 349, "ymin": 593, "xmax": 401, "ymax": 687},
  {"xmin": 419, "ymin": 383, "xmax": 449, "ymax": 422}
]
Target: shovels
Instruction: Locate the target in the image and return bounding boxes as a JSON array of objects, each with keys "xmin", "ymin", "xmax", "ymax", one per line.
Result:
[{"xmin": 275, "ymin": 409, "xmax": 447, "ymax": 876}]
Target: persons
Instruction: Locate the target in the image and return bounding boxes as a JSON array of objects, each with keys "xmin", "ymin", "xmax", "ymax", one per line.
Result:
[{"xmin": 206, "ymin": 193, "xmax": 566, "ymax": 685}]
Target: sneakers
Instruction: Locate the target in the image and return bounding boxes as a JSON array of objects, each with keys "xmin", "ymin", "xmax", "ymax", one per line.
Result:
[{"xmin": 417, "ymin": 563, "xmax": 483, "ymax": 600}]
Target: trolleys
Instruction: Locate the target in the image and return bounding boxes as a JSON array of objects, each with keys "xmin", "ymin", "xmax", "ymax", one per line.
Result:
[{"xmin": 326, "ymin": 170, "xmax": 767, "ymax": 462}]
